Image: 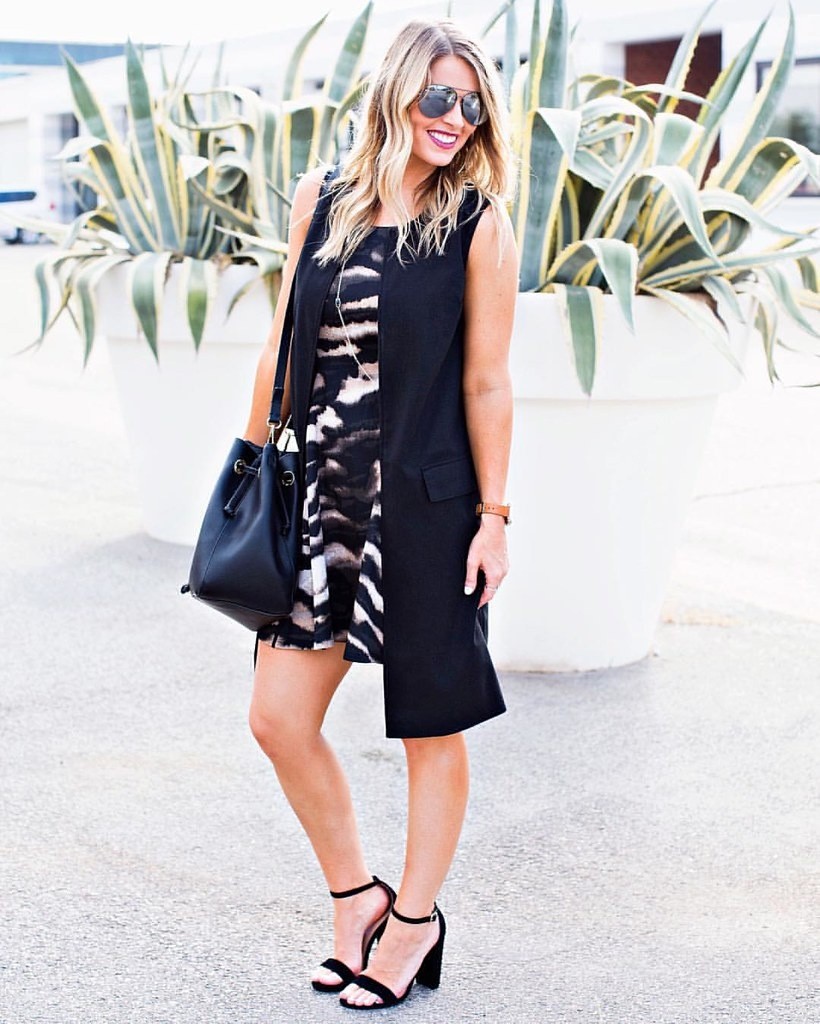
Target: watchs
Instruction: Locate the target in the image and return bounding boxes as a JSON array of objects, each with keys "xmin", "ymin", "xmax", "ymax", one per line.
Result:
[{"xmin": 475, "ymin": 501, "xmax": 512, "ymax": 526}]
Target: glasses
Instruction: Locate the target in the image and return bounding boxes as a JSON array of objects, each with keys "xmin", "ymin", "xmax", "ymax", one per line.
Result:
[{"xmin": 416, "ymin": 84, "xmax": 489, "ymax": 126}]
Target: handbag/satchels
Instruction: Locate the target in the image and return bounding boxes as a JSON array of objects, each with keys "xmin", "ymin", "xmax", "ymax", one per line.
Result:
[{"xmin": 180, "ymin": 437, "xmax": 305, "ymax": 633}]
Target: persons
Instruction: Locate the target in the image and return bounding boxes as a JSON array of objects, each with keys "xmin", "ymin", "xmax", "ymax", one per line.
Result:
[{"xmin": 241, "ymin": 18, "xmax": 520, "ymax": 1010}]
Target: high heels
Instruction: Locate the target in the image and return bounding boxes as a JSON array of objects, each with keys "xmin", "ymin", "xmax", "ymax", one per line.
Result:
[
  {"xmin": 339, "ymin": 900, "xmax": 447, "ymax": 1010},
  {"xmin": 311, "ymin": 874, "xmax": 398, "ymax": 993}
]
